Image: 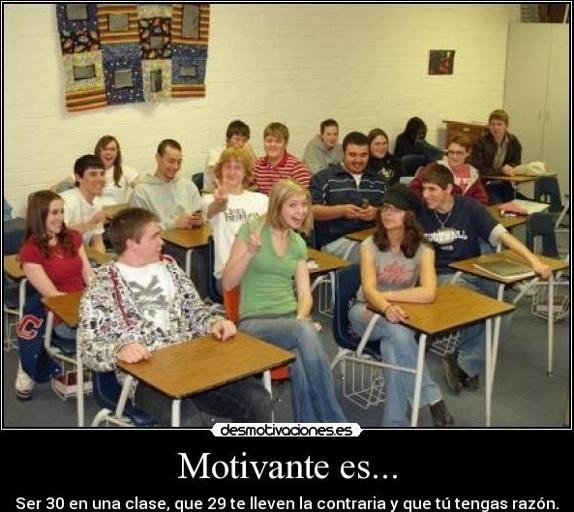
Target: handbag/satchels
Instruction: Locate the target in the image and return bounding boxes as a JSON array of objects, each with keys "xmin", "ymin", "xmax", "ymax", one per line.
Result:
[{"xmin": 497, "ymin": 199, "xmax": 549, "ymax": 218}]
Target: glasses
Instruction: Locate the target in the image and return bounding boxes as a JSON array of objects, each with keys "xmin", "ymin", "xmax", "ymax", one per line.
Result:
[{"xmin": 381, "ymin": 204, "xmax": 399, "ymax": 212}]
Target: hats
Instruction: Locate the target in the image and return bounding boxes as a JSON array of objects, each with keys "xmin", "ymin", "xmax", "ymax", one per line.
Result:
[{"xmin": 383, "ymin": 183, "xmax": 422, "ymax": 217}]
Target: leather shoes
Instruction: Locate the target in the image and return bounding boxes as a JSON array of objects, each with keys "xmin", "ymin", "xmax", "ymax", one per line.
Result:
[
  {"xmin": 429, "ymin": 400, "xmax": 453, "ymax": 427},
  {"xmin": 452, "ymin": 347, "xmax": 478, "ymax": 392},
  {"xmin": 441, "ymin": 354, "xmax": 462, "ymax": 394}
]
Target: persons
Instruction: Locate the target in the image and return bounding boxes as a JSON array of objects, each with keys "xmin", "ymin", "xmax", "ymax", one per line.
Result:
[
  {"xmin": 128, "ymin": 139, "xmax": 203, "ymax": 236},
  {"xmin": 94, "ymin": 135, "xmax": 140, "ymax": 187},
  {"xmin": 222, "ymin": 180, "xmax": 348, "ymax": 423},
  {"xmin": 306, "ymin": 110, "xmax": 560, "ymax": 427},
  {"xmin": 77, "ymin": 208, "xmax": 272, "ymax": 428},
  {"xmin": 20, "ymin": 190, "xmax": 97, "ymax": 341},
  {"xmin": 203, "ymin": 118, "xmax": 258, "ymax": 193},
  {"xmin": 200, "ymin": 146, "xmax": 271, "ymax": 298},
  {"xmin": 244, "ymin": 122, "xmax": 311, "ymax": 196},
  {"xmin": 56, "ymin": 155, "xmax": 118, "ymax": 248}
]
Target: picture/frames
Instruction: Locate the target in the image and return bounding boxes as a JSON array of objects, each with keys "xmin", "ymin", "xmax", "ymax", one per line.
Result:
[{"xmin": 429, "ymin": 49, "xmax": 455, "ymax": 74}]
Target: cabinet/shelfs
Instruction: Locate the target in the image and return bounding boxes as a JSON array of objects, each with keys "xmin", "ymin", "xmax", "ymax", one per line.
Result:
[
  {"xmin": 444, "ymin": 118, "xmax": 490, "ymax": 157},
  {"xmin": 501, "ymin": 22, "xmax": 571, "ymax": 204}
]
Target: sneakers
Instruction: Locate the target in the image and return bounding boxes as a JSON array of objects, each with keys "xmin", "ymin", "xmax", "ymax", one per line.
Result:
[{"xmin": 14, "ymin": 360, "xmax": 35, "ymax": 399}]
